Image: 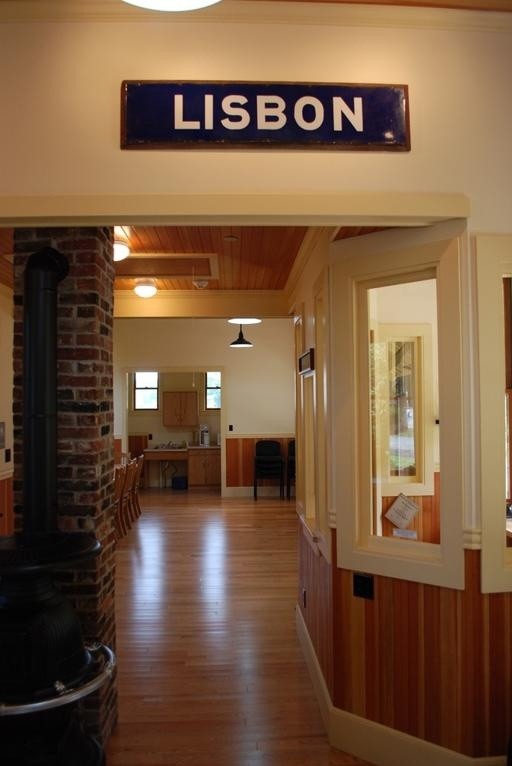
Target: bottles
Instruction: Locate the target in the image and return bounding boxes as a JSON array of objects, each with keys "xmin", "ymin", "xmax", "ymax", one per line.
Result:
[{"xmin": 216, "ymin": 431, "xmax": 220, "ymax": 445}]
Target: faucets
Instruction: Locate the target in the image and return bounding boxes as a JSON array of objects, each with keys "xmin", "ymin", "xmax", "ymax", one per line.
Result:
[{"xmin": 167, "ymin": 440, "xmax": 172, "ymax": 445}]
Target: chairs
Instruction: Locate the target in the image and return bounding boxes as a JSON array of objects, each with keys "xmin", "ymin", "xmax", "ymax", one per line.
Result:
[
  {"xmin": 113, "ymin": 453, "xmax": 145, "ymax": 544},
  {"xmin": 287, "ymin": 439, "xmax": 302, "ymax": 501},
  {"xmin": 254, "ymin": 441, "xmax": 284, "ymax": 500}
]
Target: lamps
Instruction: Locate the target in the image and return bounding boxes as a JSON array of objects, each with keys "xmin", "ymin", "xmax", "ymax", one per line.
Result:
[
  {"xmin": 134, "ymin": 284, "xmax": 157, "ymax": 298},
  {"xmin": 230, "ymin": 324, "xmax": 253, "ymax": 348},
  {"xmin": 227, "ymin": 318, "xmax": 261, "ymax": 324},
  {"xmin": 113, "ymin": 241, "xmax": 130, "ymax": 262}
]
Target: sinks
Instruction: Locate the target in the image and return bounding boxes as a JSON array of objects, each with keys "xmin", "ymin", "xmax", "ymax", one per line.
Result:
[
  {"xmin": 153, "ymin": 444, "xmax": 167, "ymax": 448},
  {"xmin": 163, "ymin": 444, "xmax": 186, "ymax": 449}
]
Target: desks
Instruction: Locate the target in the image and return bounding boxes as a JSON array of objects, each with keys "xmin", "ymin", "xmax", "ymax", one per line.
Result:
[{"xmin": 0, "ymin": 529, "xmax": 116, "ymax": 766}]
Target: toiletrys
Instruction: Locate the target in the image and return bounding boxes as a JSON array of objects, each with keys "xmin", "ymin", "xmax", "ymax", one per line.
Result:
[{"xmin": 203, "ymin": 432, "xmax": 210, "ymax": 446}]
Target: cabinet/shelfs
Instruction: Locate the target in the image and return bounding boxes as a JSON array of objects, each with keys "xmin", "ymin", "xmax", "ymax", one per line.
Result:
[{"xmin": 127, "ymin": 391, "xmax": 221, "ymax": 491}]
